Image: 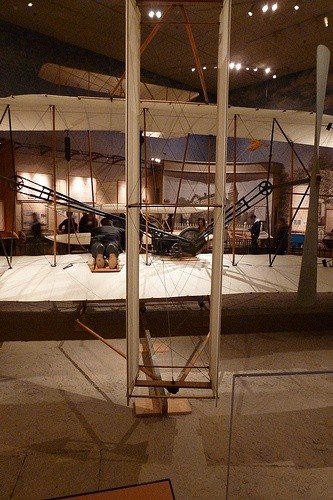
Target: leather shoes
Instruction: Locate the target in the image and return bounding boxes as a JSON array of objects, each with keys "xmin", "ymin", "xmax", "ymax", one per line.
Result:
[
  {"xmin": 106, "ymin": 244, "xmax": 118, "ymax": 269},
  {"xmin": 95, "ymin": 244, "xmax": 105, "ymax": 269}
]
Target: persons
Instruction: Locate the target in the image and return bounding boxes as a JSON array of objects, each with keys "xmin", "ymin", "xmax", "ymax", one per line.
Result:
[
  {"xmin": 58, "ymin": 212, "xmax": 77, "ymax": 250},
  {"xmin": 248, "ymin": 214, "xmax": 260, "ymax": 254},
  {"xmin": 31, "ymin": 212, "xmax": 41, "ymax": 237},
  {"xmin": 79, "ymin": 213, "xmax": 98, "ymax": 251},
  {"xmin": 197, "ymin": 217, "xmax": 209, "ymax": 254},
  {"xmin": 91, "ymin": 219, "xmax": 126, "ymax": 269},
  {"xmin": 278, "ymin": 218, "xmax": 289, "ymax": 255}
]
